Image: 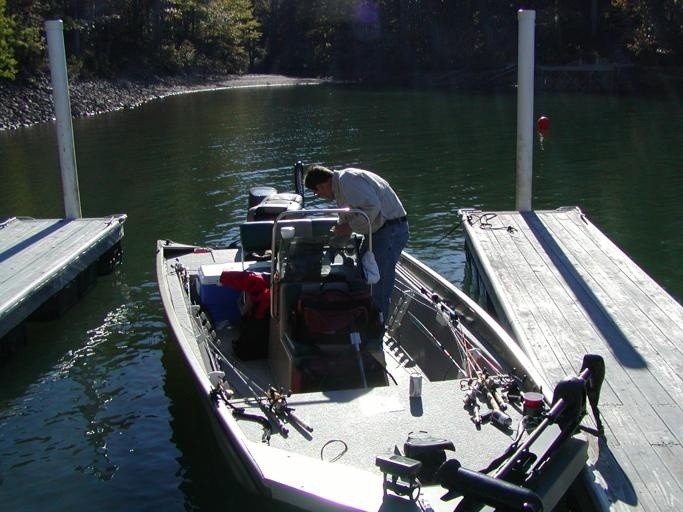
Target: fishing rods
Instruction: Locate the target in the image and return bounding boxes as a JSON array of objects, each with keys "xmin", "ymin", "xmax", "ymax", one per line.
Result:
[
  {"xmin": 185, "ymin": 280, "xmax": 316, "ymax": 437},
  {"xmin": 396, "ymin": 261, "xmax": 537, "ymax": 430}
]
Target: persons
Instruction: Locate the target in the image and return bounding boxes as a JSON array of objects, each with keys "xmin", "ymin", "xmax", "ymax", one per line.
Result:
[{"xmin": 304, "ymin": 164, "xmax": 410, "ymax": 331}]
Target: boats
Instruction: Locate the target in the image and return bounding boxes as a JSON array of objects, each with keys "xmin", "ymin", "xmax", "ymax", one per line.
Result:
[{"xmin": 154, "ymin": 177, "xmax": 601, "ymax": 511}]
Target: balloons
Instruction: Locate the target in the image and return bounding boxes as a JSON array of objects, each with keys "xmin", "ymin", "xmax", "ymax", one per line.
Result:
[{"xmin": 538, "ymin": 116, "xmax": 550, "ymax": 136}]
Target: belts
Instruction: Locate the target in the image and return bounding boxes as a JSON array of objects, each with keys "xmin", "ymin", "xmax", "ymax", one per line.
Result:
[{"xmin": 386, "ymin": 215, "xmax": 408, "ymax": 225}]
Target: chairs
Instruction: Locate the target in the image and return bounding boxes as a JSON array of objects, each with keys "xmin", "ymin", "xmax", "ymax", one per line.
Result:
[{"xmin": 270, "ymin": 208, "xmax": 387, "ymax": 388}]
